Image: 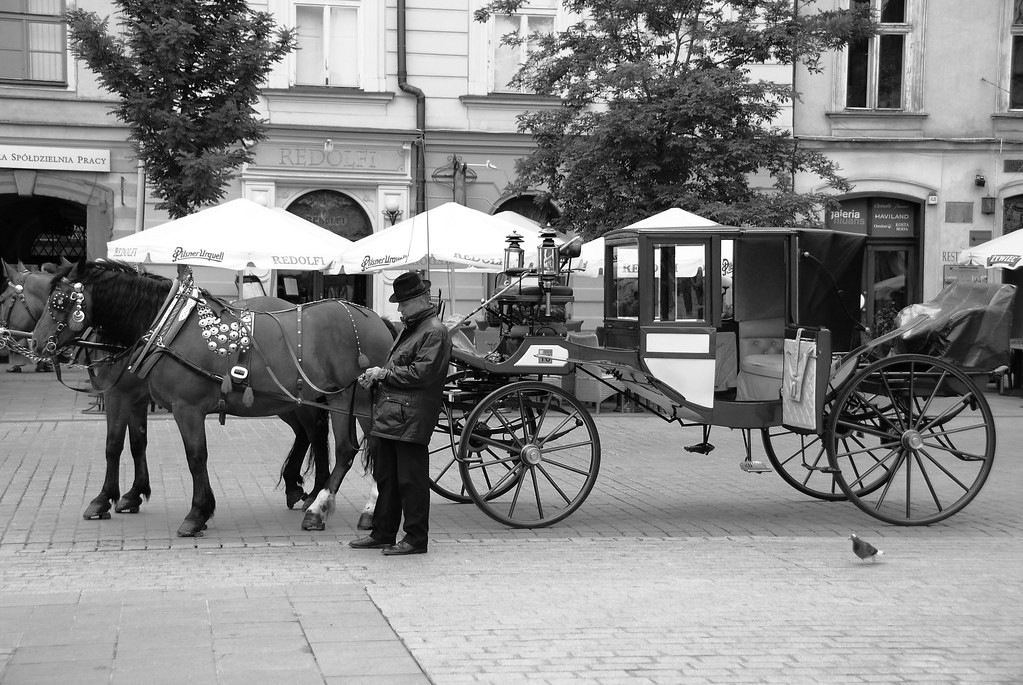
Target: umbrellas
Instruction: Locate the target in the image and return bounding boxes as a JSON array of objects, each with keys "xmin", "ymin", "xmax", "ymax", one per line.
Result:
[
  {"xmin": 106, "ymin": 198, "xmax": 732, "ymax": 320},
  {"xmin": 956, "ymin": 228, "xmax": 1023, "ymax": 270}
]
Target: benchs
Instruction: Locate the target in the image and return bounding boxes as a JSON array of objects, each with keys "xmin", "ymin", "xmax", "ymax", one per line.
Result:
[
  {"xmin": 736, "ymin": 319, "xmax": 836, "ymax": 399},
  {"xmin": 891, "ymin": 281, "xmax": 1017, "ymax": 373},
  {"xmin": 492, "ymin": 242, "xmax": 583, "ymax": 305}
]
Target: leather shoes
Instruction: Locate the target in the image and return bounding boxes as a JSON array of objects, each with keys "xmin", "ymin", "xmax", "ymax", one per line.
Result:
[
  {"xmin": 348, "ymin": 534, "xmax": 394, "ymax": 549},
  {"xmin": 380, "ymin": 541, "xmax": 428, "ymax": 555}
]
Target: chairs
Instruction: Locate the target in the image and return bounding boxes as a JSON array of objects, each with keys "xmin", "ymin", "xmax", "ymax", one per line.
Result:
[{"xmin": 459, "ymin": 314, "xmax": 625, "ymax": 415}]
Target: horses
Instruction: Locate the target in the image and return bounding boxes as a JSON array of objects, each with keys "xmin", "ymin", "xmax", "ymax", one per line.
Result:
[
  {"xmin": 0, "ymin": 256, "xmax": 331, "ymax": 521},
  {"xmin": 27, "ymin": 257, "xmax": 401, "ymax": 539}
]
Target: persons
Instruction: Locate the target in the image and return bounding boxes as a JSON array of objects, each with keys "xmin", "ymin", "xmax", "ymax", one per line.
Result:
[
  {"xmin": 34, "ymin": 262, "xmax": 56, "ymax": 372},
  {"xmin": 348, "ymin": 272, "xmax": 454, "ymax": 555},
  {"xmin": 681, "ymin": 278, "xmax": 692, "ymax": 314},
  {"xmin": 692, "ymin": 267, "xmax": 703, "ymax": 318}
]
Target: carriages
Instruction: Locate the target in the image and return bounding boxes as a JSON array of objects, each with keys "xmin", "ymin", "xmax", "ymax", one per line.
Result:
[{"xmin": 0, "ymin": 224, "xmax": 1016, "ymax": 539}]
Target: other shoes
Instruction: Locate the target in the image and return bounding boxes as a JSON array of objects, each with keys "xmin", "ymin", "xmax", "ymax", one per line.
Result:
[
  {"xmin": 6, "ymin": 367, "xmax": 22, "ymax": 373},
  {"xmin": 34, "ymin": 362, "xmax": 54, "ymax": 372}
]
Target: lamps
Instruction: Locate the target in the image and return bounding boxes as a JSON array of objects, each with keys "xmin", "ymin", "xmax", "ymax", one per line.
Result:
[
  {"xmin": 975, "ymin": 174, "xmax": 985, "ymax": 188},
  {"xmin": 382, "ymin": 198, "xmax": 409, "ymax": 224},
  {"xmin": 981, "ymin": 195, "xmax": 995, "ymax": 216},
  {"xmin": 323, "ymin": 135, "xmax": 335, "ymax": 151}
]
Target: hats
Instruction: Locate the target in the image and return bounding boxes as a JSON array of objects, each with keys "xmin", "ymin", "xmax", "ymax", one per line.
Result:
[{"xmin": 389, "ymin": 272, "xmax": 431, "ymax": 303}]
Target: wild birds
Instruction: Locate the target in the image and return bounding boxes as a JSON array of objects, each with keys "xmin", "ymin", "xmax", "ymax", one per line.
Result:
[{"xmin": 847, "ymin": 533, "xmax": 886, "ymax": 563}]
